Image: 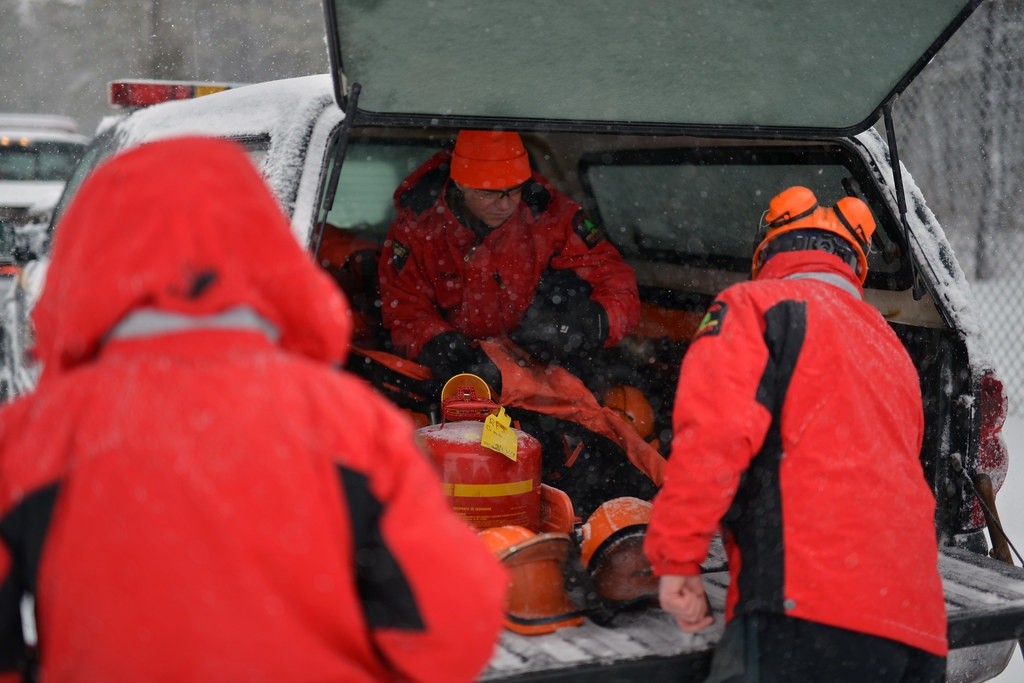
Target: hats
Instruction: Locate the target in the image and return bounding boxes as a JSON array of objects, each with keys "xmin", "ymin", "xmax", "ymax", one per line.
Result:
[{"xmin": 449, "ymin": 128, "xmax": 532, "ymax": 189}]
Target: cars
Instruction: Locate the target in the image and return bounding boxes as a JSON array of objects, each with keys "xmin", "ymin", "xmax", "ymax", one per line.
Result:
[
  {"xmin": 1, "ymin": 124, "xmax": 95, "ymax": 279},
  {"xmin": 1, "ymin": 0, "xmax": 1024, "ymax": 683}
]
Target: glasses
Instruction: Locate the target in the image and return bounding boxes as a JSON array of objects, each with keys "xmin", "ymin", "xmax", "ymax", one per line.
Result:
[{"xmin": 464, "ymin": 186, "xmax": 525, "ymax": 199}]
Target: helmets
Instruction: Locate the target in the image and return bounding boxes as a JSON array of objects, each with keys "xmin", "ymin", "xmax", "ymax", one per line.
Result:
[
  {"xmin": 591, "ymin": 534, "xmax": 664, "ymax": 604},
  {"xmin": 751, "ymin": 185, "xmax": 877, "ymax": 284},
  {"xmin": 475, "ymin": 524, "xmax": 573, "ymax": 569},
  {"xmin": 503, "ymin": 562, "xmax": 587, "ymax": 635},
  {"xmin": 604, "ymin": 385, "xmax": 659, "ymax": 453},
  {"xmin": 578, "ymin": 497, "xmax": 661, "ymax": 574}
]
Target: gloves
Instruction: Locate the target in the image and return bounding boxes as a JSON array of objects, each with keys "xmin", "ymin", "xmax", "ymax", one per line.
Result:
[
  {"xmin": 418, "ymin": 329, "xmax": 502, "ymax": 396},
  {"xmin": 544, "ymin": 299, "xmax": 609, "ymax": 361}
]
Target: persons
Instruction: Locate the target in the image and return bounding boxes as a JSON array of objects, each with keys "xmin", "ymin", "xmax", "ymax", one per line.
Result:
[
  {"xmin": 642, "ymin": 186, "xmax": 950, "ymax": 683},
  {"xmin": 0, "ymin": 134, "xmax": 511, "ymax": 683},
  {"xmin": 375, "ymin": 130, "xmax": 642, "ymax": 476}
]
